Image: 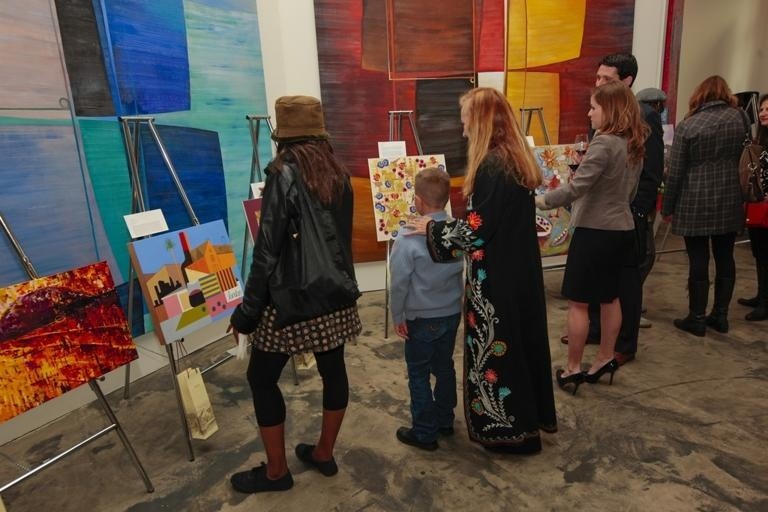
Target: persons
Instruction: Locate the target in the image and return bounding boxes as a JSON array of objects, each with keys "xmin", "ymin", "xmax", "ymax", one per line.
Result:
[
  {"xmin": 737, "ymin": 94, "xmax": 768, "ymax": 321},
  {"xmin": 636, "ymin": 88, "xmax": 668, "ymax": 330},
  {"xmin": 534, "ymin": 80, "xmax": 653, "ymax": 397},
  {"xmin": 559, "ymin": 51, "xmax": 664, "ymax": 367},
  {"xmin": 660, "ymin": 75, "xmax": 751, "ymax": 337},
  {"xmin": 226, "ymin": 96, "xmax": 363, "ymax": 494},
  {"xmin": 388, "ymin": 168, "xmax": 464, "ymax": 452},
  {"xmin": 401, "ymin": 86, "xmax": 557, "ymax": 455}
]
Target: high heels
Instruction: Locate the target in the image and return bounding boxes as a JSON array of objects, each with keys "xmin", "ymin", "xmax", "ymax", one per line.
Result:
[
  {"xmin": 584, "ymin": 357, "xmax": 620, "ymax": 386},
  {"xmin": 556, "ymin": 368, "xmax": 584, "ymax": 395}
]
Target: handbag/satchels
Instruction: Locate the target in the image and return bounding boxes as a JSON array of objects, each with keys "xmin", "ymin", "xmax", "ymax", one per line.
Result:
[
  {"xmin": 738, "ymin": 143, "xmax": 768, "ymax": 202},
  {"xmin": 265, "ymin": 151, "xmax": 359, "ymax": 324}
]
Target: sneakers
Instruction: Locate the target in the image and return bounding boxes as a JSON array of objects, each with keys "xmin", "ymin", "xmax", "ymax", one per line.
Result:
[
  {"xmin": 294, "ymin": 443, "xmax": 338, "ymax": 478},
  {"xmin": 672, "ymin": 308, "xmax": 728, "ymax": 338},
  {"xmin": 738, "ymin": 295, "xmax": 768, "ymax": 322},
  {"xmin": 614, "ymin": 351, "xmax": 636, "ymax": 367},
  {"xmin": 231, "ymin": 460, "xmax": 294, "ymax": 492},
  {"xmin": 397, "ymin": 419, "xmax": 455, "ymax": 452}
]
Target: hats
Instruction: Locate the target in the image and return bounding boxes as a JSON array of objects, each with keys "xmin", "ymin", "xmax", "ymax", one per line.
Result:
[
  {"xmin": 636, "ymin": 86, "xmax": 667, "ymax": 103},
  {"xmin": 271, "ymin": 95, "xmax": 333, "ymax": 142}
]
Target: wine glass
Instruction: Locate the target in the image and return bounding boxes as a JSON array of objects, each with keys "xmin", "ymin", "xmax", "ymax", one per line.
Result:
[{"xmin": 566, "ymin": 134, "xmax": 588, "ymax": 177}]
